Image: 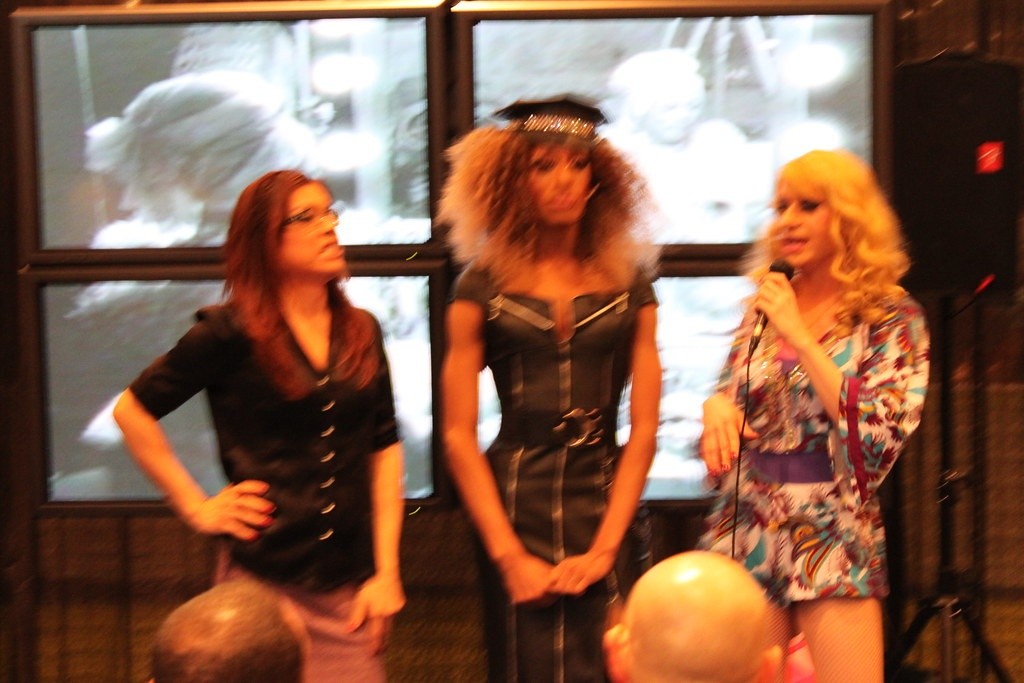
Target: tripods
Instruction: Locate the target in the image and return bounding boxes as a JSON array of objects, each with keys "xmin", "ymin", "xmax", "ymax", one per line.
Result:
[{"xmin": 880, "ymin": 283, "xmax": 1021, "ymax": 683}]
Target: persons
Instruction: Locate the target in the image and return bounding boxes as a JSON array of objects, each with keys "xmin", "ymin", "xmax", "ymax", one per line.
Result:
[
  {"xmin": 113, "ymin": 169, "xmax": 405, "ymax": 682},
  {"xmin": 430, "ymin": 96, "xmax": 660, "ymax": 683},
  {"xmin": 151, "ymin": 578, "xmax": 304, "ymax": 683},
  {"xmin": 603, "ymin": 550, "xmax": 764, "ymax": 683},
  {"xmin": 699, "ymin": 150, "xmax": 929, "ymax": 683}
]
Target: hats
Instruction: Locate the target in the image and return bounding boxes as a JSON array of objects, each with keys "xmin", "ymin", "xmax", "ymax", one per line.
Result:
[{"xmin": 490, "ymin": 96, "xmax": 610, "ymax": 133}]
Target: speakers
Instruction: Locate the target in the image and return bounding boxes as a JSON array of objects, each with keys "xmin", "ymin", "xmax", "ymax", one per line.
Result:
[{"xmin": 884, "ymin": 53, "xmax": 1022, "ymax": 291}]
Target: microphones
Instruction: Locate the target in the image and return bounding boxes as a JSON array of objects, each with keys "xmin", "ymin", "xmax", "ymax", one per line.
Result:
[{"xmin": 751, "ymin": 256, "xmax": 796, "ymax": 346}]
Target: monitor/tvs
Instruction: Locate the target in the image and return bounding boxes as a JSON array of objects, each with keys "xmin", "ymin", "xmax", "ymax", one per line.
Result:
[{"xmin": 4, "ymin": 3, "xmax": 888, "ymax": 520}]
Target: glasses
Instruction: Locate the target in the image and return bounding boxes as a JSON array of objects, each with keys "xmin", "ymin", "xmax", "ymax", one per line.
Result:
[{"xmin": 281, "ymin": 205, "xmax": 341, "ymax": 229}]
